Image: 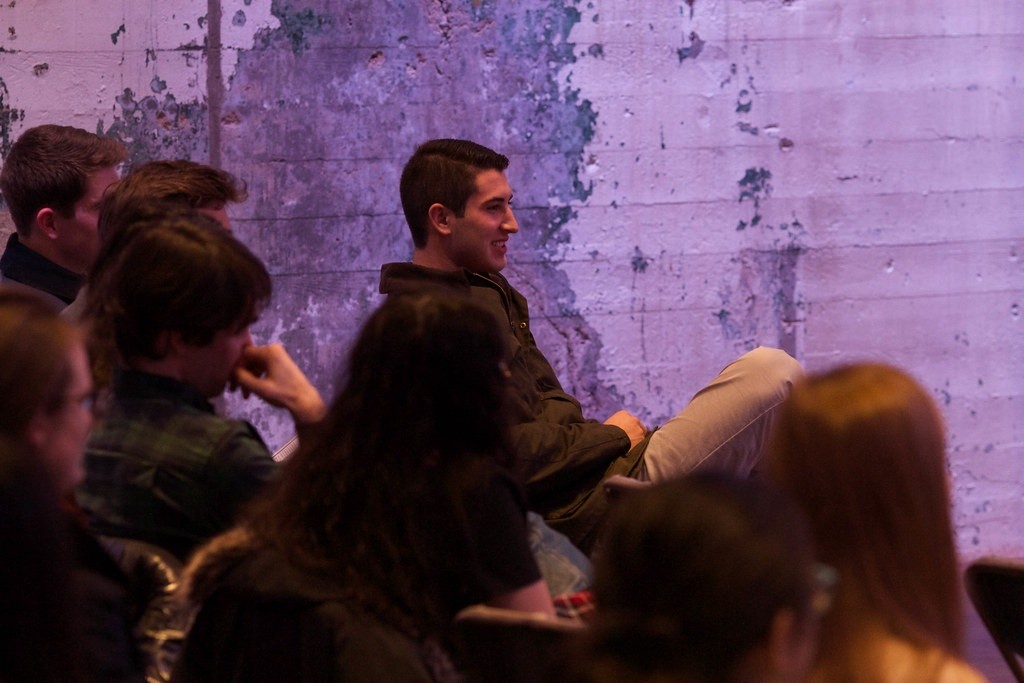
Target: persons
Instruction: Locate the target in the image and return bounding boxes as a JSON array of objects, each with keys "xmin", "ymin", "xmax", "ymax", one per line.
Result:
[{"xmin": 0, "ymin": 124, "xmax": 990, "ymax": 683}]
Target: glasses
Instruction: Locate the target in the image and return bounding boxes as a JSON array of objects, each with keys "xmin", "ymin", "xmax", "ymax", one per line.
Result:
[
  {"xmin": 49, "ymin": 382, "xmax": 113, "ymax": 419},
  {"xmin": 807, "ymin": 563, "xmax": 839, "ymax": 612}
]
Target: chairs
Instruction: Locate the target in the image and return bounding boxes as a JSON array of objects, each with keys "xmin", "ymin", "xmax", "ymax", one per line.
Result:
[
  {"xmin": 449, "ymin": 605, "xmax": 592, "ymax": 683},
  {"xmin": 963, "ymin": 556, "xmax": 1024, "ymax": 662},
  {"xmin": 95, "ymin": 534, "xmax": 196, "ymax": 683}
]
